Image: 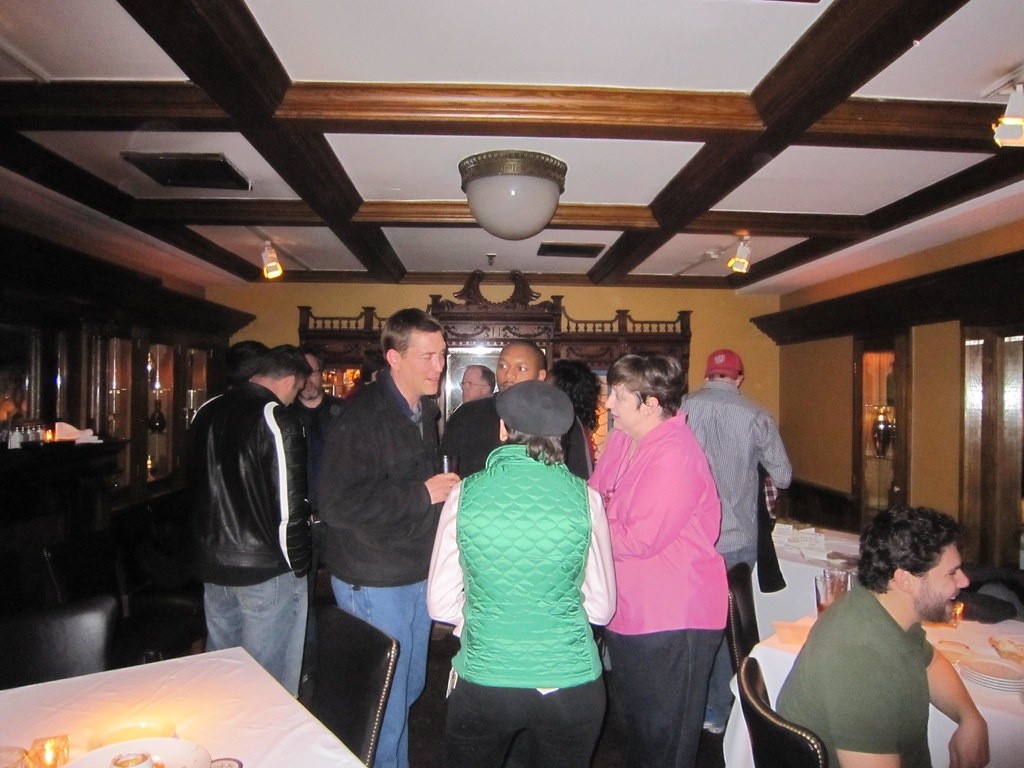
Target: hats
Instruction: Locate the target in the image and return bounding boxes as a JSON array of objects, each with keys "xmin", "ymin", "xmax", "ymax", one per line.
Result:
[
  {"xmin": 704, "ymin": 349, "xmax": 744, "ymax": 379},
  {"xmin": 494, "ymin": 379, "xmax": 574, "ymax": 436}
]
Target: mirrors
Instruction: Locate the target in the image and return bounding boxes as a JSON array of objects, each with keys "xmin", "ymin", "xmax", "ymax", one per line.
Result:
[{"xmin": 444, "ymin": 346, "xmax": 547, "ymax": 417}]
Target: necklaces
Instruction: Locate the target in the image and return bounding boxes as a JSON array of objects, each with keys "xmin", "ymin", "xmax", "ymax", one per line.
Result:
[{"xmin": 602, "ymin": 437, "xmax": 633, "ymax": 509}]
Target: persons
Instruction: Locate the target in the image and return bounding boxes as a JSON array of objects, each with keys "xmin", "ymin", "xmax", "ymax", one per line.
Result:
[
  {"xmin": 775, "ymin": 503, "xmax": 990, "ymax": 768},
  {"xmin": 190, "ymin": 340, "xmax": 270, "ymax": 439},
  {"xmin": 545, "ymin": 358, "xmax": 602, "ymax": 462},
  {"xmin": 440, "ymin": 339, "xmax": 592, "ymax": 480},
  {"xmin": 426, "ymin": 380, "xmax": 617, "ymax": 768},
  {"xmin": 587, "ymin": 351, "xmax": 729, "ymax": 768},
  {"xmin": 289, "ymin": 346, "xmax": 345, "ymax": 697},
  {"xmin": 458, "ymin": 365, "xmax": 496, "ymax": 402},
  {"xmin": 680, "ymin": 348, "xmax": 793, "ymax": 733},
  {"xmin": 351, "ymin": 350, "xmax": 386, "ymax": 393},
  {"xmin": 191, "ymin": 344, "xmax": 313, "ymax": 700},
  {"xmin": 317, "ymin": 308, "xmax": 461, "ymax": 768}
]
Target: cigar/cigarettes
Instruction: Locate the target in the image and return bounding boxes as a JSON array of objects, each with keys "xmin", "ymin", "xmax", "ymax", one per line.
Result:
[{"xmin": 443, "ymin": 455, "xmax": 447, "ymax": 474}]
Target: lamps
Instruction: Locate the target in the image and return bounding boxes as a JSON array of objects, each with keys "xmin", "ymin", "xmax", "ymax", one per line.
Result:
[
  {"xmin": 727, "ymin": 235, "xmax": 753, "ymax": 272},
  {"xmin": 262, "ymin": 241, "xmax": 283, "ymax": 279},
  {"xmin": 458, "ymin": 150, "xmax": 568, "ymax": 241},
  {"xmin": 992, "ymin": 81, "xmax": 1024, "ymax": 148}
]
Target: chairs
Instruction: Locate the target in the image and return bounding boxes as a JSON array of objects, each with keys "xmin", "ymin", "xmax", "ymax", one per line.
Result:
[
  {"xmin": 304, "ymin": 606, "xmax": 399, "ymax": 768},
  {"xmin": 724, "ymin": 561, "xmax": 829, "ymax": 767},
  {"xmin": 0, "ymin": 482, "xmax": 209, "ymax": 693}
]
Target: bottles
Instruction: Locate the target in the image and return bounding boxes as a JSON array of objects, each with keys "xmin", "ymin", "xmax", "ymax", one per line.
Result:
[
  {"xmin": 8, "ymin": 425, "xmax": 47, "ymax": 449},
  {"xmin": 149, "ymin": 400, "xmax": 166, "ymax": 434}
]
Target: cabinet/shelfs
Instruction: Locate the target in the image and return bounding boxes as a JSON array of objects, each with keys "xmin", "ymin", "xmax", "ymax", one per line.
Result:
[{"xmin": 0, "ymin": 224, "xmax": 247, "ymax": 607}]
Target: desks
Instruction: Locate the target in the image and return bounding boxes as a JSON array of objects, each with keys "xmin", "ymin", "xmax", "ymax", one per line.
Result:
[
  {"xmin": 721, "ymin": 615, "xmax": 1024, "ymax": 768},
  {"xmin": 0, "ymin": 645, "xmax": 369, "ymax": 768},
  {"xmin": 751, "ymin": 524, "xmax": 861, "ymax": 642}
]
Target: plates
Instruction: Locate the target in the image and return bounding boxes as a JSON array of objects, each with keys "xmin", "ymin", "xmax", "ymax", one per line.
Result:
[
  {"xmin": 957, "ymin": 654, "xmax": 1024, "ymax": 693},
  {"xmin": 64, "ymin": 737, "xmax": 212, "ymax": 768}
]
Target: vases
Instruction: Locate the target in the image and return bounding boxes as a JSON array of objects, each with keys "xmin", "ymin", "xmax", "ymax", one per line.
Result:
[{"xmin": 871, "ymin": 405, "xmax": 893, "ymax": 457}]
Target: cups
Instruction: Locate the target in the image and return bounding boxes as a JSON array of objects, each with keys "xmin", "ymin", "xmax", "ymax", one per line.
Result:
[
  {"xmin": 110, "ymin": 750, "xmax": 164, "ymax": 768},
  {"xmin": 824, "ymin": 568, "xmax": 849, "ymax": 602},
  {"xmin": 814, "ymin": 575, "xmax": 840, "ymax": 615},
  {"xmin": 437, "ymin": 454, "xmax": 459, "ymax": 474},
  {"xmin": 0, "ymin": 735, "xmax": 72, "ymax": 768}
]
